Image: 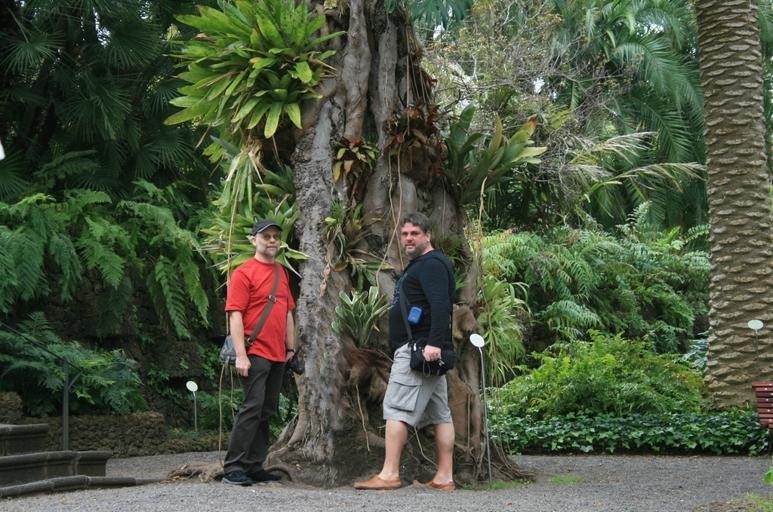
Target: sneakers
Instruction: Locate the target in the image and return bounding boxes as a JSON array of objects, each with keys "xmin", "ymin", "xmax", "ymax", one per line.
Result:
[
  {"xmin": 354, "ymin": 474, "xmax": 401, "ymax": 490},
  {"xmin": 413, "ymin": 477, "xmax": 456, "ymax": 491},
  {"xmin": 252, "ymin": 467, "xmax": 282, "ymax": 483},
  {"xmin": 223, "ymin": 471, "xmax": 252, "ymax": 486}
]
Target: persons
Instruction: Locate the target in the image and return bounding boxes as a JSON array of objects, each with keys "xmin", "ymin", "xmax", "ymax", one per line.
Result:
[
  {"xmin": 354, "ymin": 212, "xmax": 457, "ymax": 493},
  {"xmin": 220, "ymin": 221, "xmax": 296, "ymax": 486}
]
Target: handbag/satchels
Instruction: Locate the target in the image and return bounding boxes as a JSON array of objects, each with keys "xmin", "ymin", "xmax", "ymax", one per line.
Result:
[
  {"xmin": 218, "ymin": 333, "xmax": 252, "ymax": 365},
  {"xmin": 409, "ymin": 342, "xmax": 456, "ymax": 376}
]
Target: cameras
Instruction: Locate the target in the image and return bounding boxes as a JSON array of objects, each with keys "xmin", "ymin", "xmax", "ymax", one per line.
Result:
[{"xmin": 285, "ymin": 354, "xmax": 305, "ymax": 375}]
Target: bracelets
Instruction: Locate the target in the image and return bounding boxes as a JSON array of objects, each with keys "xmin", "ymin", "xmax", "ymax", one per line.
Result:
[{"xmin": 286, "ymin": 348, "xmax": 294, "ymax": 352}]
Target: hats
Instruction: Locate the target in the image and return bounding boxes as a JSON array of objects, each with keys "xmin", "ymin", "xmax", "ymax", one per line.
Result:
[{"xmin": 252, "ymin": 220, "xmax": 283, "ymax": 236}]
